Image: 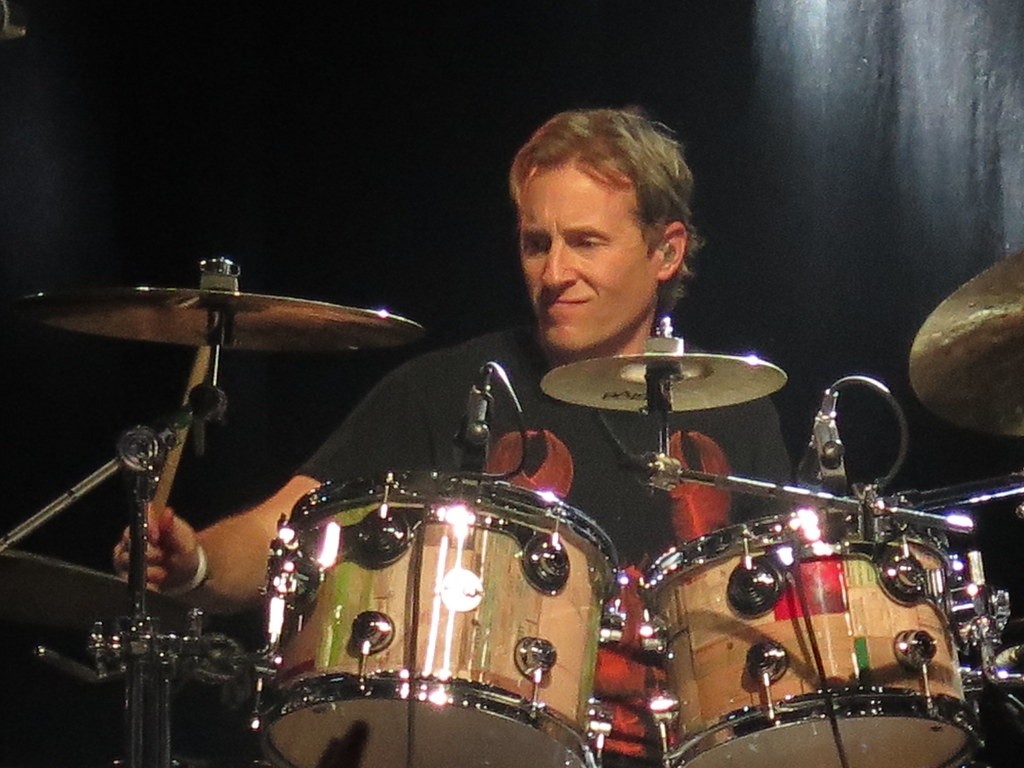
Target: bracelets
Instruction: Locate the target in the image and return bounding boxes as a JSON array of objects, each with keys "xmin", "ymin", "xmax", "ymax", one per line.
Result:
[{"xmin": 144, "ymin": 536, "xmax": 206, "ymax": 597}]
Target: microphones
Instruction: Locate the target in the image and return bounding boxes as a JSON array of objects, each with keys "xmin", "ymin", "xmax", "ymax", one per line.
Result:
[
  {"xmin": 794, "ymin": 388, "xmax": 839, "ymax": 491},
  {"xmin": 454, "ymin": 366, "xmax": 492, "ymax": 468}
]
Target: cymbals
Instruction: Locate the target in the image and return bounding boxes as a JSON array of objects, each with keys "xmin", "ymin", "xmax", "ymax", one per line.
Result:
[
  {"xmin": 908, "ymin": 251, "xmax": 1024, "ymax": 438},
  {"xmin": 14, "ymin": 286, "xmax": 427, "ymax": 348},
  {"xmin": 0, "ymin": 547, "xmax": 195, "ymax": 620},
  {"xmin": 541, "ymin": 352, "xmax": 788, "ymax": 413}
]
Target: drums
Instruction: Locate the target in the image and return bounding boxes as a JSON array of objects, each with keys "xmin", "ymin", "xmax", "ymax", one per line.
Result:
[
  {"xmin": 636, "ymin": 506, "xmax": 985, "ymax": 768},
  {"xmin": 252, "ymin": 470, "xmax": 620, "ymax": 768}
]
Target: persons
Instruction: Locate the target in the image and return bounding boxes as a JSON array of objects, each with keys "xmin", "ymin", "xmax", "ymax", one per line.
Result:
[{"xmin": 114, "ymin": 106, "xmax": 794, "ymax": 767}]
